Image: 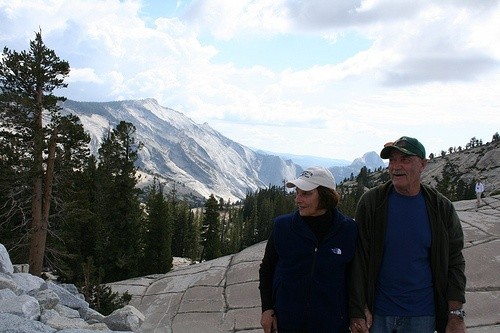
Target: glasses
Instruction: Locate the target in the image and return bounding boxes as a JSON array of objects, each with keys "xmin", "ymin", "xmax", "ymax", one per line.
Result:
[{"xmin": 384, "ymin": 139, "xmax": 423, "ymax": 159}]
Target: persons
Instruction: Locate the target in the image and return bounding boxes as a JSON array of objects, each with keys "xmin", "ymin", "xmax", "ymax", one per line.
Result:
[
  {"xmin": 475, "ymin": 180, "xmax": 484, "ymax": 204},
  {"xmin": 257, "ymin": 164, "xmax": 373, "ymax": 333},
  {"xmin": 353, "ymin": 135, "xmax": 466, "ymax": 333}
]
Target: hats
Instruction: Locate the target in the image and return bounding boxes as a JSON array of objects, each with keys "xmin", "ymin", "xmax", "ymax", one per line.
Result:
[
  {"xmin": 380, "ymin": 135, "xmax": 425, "ymax": 159},
  {"xmin": 286, "ymin": 166, "xmax": 336, "ymax": 190}
]
